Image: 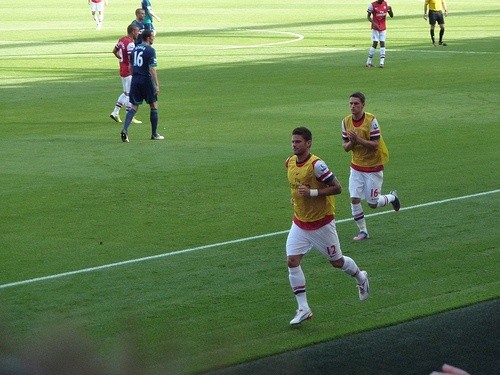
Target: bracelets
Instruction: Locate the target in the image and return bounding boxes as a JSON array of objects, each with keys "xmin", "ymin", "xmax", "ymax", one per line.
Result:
[
  {"xmin": 309, "ymin": 188, "xmax": 318, "ymax": 197},
  {"xmin": 425, "ymin": 13, "xmax": 428, "ymax": 16}
]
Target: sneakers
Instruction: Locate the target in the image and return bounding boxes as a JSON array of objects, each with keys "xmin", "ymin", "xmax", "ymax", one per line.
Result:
[
  {"xmin": 367, "ymin": 58, "xmax": 372, "ymax": 68},
  {"xmin": 439, "ymin": 41, "xmax": 447, "ymax": 46},
  {"xmin": 353, "ymin": 231, "xmax": 369, "ymax": 240},
  {"xmin": 152, "ymin": 134, "xmax": 164, "ymax": 139},
  {"xmin": 379, "ymin": 59, "xmax": 384, "ymax": 68},
  {"xmin": 131, "ymin": 117, "xmax": 142, "ymax": 123},
  {"xmin": 120, "ymin": 128, "xmax": 129, "ymax": 142},
  {"xmin": 432, "ymin": 42, "xmax": 436, "ymax": 48},
  {"xmin": 290, "ymin": 305, "xmax": 312, "ymax": 325},
  {"xmin": 110, "ymin": 112, "xmax": 122, "ymax": 122},
  {"xmin": 390, "ymin": 190, "xmax": 400, "ymax": 211},
  {"xmin": 356, "ymin": 271, "xmax": 369, "ymax": 300}
]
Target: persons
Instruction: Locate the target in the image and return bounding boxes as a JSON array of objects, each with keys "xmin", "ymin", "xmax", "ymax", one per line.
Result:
[
  {"xmin": 445, "ymin": 10, "xmax": 447, "ymax": 13},
  {"xmin": 130, "ymin": 8, "xmax": 145, "ymax": 46},
  {"xmin": 424, "ymin": 0, "xmax": 448, "ymax": 48},
  {"xmin": 341, "ymin": 91, "xmax": 400, "ymax": 241},
  {"xmin": 88, "ymin": 0, "xmax": 108, "ymax": 31},
  {"xmin": 110, "ymin": 24, "xmax": 143, "ymax": 124},
  {"xmin": 367, "ymin": 0, "xmax": 393, "ymax": 68},
  {"xmin": 141, "ymin": 0, "xmax": 160, "ymax": 37},
  {"xmin": 120, "ymin": 29, "xmax": 165, "ymax": 142},
  {"xmin": 286, "ymin": 126, "xmax": 369, "ymax": 325}
]
